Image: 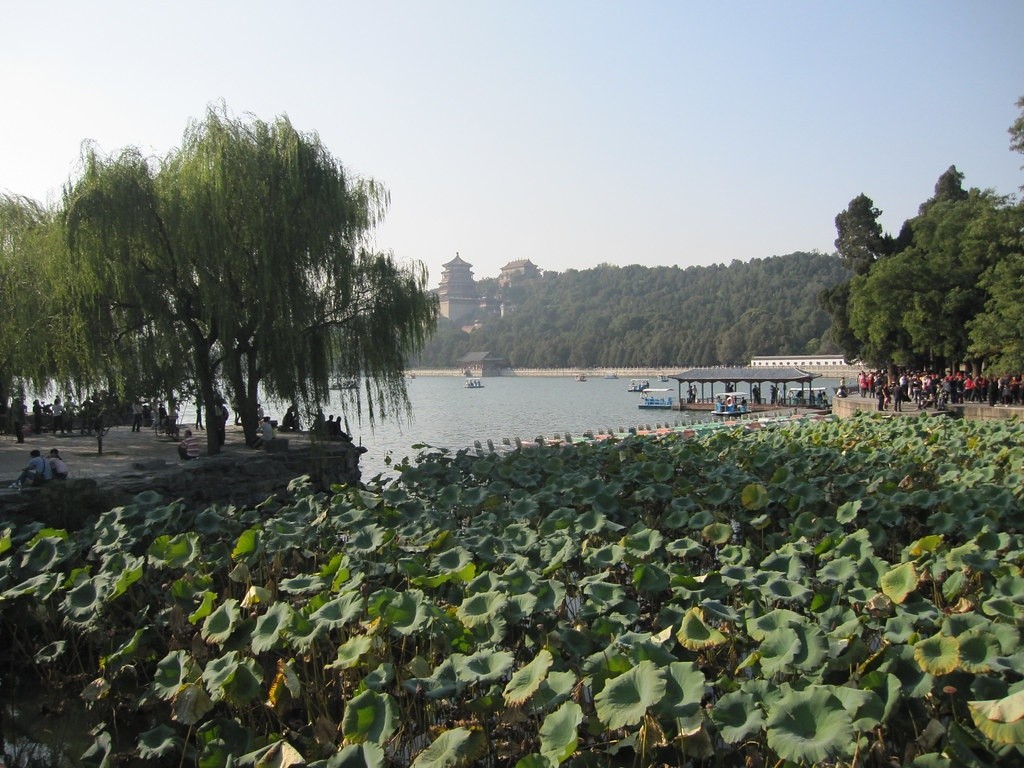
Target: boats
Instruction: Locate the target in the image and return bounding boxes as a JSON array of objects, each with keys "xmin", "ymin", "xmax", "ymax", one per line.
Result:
[
  {"xmin": 657, "ymin": 373, "xmax": 670, "ymax": 383},
  {"xmin": 574, "ymin": 374, "xmax": 587, "ymax": 382},
  {"xmin": 711, "ymin": 391, "xmax": 752, "ymax": 417},
  {"xmin": 603, "ymin": 372, "xmax": 619, "ymax": 380},
  {"xmin": 627, "ymin": 378, "xmax": 650, "ymax": 393},
  {"xmin": 783, "ymin": 386, "xmax": 828, "ymax": 410},
  {"xmin": 636, "ymin": 388, "xmax": 674, "ymax": 410},
  {"xmin": 464, "ymin": 378, "xmax": 485, "ymax": 389}
]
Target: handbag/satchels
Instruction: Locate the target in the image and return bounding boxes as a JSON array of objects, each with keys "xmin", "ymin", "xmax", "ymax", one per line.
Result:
[{"xmin": 35, "ymin": 472, "xmax": 44, "ymax": 482}]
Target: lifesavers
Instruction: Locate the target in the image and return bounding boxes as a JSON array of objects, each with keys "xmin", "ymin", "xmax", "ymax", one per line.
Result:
[
  {"xmin": 640, "ymin": 392, "xmax": 648, "ymax": 399},
  {"xmin": 787, "ymin": 391, "xmax": 794, "ymax": 398},
  {"xmin": 725, "ymin": 396, "xmax": 734, "ymax": 407}
]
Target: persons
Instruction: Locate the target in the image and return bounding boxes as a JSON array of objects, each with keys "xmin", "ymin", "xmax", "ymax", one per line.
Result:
[
  {"xmin": 283, "ymin": 406, "xmax": 297, "ymax": 431},
  {"xmin": 9, "ymin": 398, "xmax": 27, "ymax": 444},
  {"xmin": 130, "ymin": 397, "xmax": 170, "ymax": 433},
  {"xmin": 726, "ymin": 382, "xmax": 734, "ymax": 392},
  {"xmin": 686, "ymin": 385, "xmax": 698, "ymax": 403},
  {"xmin": 51, "ymin": 393, "xmax": 93, "ymax": 436},
  {"xmin": 216, "ymin": 401, "xmax": 229, "ymax": 447},
  {"xmin": 325, "ymin": 414, "xmax": 354, "ymax": 442},
  {"xmin": 753, "ymin": 385, "xmax": 830, "ymax": 406},
  {"xmin": 856, "ymin": 369, "xmax": 1024, "ymax": 412},
  {"xmin": 741, "ymin": 397, "xmax": 747, "ymax": 404},
  {"xmin": 9, "ymin": 450, "xmax": 53, "ymax": 490},
  {"xmin": 178, "ymin": 430, "xmax": 201, "ymax": 460},
  {"xmin": 307, "ymin": 409, "xmax": 325, "ymax": 432},
  {"xmin": 256, "ymin": 403, "xmax": 264, "ymax": 420},
  {"xmin": 31, "ymin": 399, "xmax": 43, "ymax": 433},
  {"xmin": 251, "ymin": 417, "xmax": 277, "ymax": 448},
  {"xmin": 840, "ymin": 376, "xmax": 846, "ymax": 396},
  {"xmin": 47, "ymin": 449, "xmax": 68, "ymax": 479},
  {"xmin": 193, "ymin": 396, "xmax": 206, "ymax": 431},
  {"xmin": 231, "ymin": 396, "xmax": 241, "ymax": 425},
  {"xmin": 716, "ymin": 396, "xmax": 722, "ymax": 405}
]
